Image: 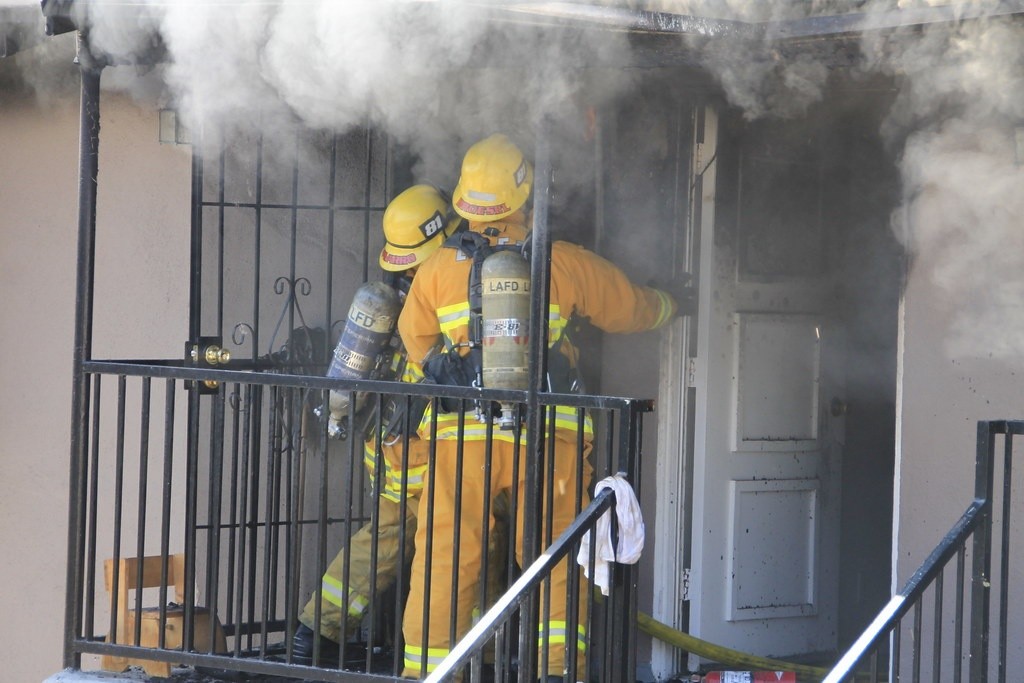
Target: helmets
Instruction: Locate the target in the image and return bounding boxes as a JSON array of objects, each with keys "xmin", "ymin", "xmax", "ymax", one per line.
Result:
[
  {"xmin": 451, "ymin": 134, "xmax": 532, "ymax": 224},
  {"xmin": 379, "ymin": 183, "xmax": 460, "ymax": 271}
]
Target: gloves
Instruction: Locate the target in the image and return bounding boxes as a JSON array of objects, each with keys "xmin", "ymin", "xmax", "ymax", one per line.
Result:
[{"xmin": 645, "ymin": 270, "xmax": 698, "ymax": 317}]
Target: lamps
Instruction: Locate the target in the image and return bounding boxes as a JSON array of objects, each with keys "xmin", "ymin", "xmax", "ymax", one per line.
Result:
[{"xmin": 156, "ymin": 96, "xmax": 191, "ymax": 145}]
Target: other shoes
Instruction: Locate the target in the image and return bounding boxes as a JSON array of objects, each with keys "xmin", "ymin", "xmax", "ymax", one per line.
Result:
[{"xmin": 292, "ymin": 630, "xmax": 367, "ymax": 667}]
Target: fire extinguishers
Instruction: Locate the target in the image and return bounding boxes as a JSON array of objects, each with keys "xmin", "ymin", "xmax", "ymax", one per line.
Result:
[{"xmin": 690, "ymin": 670, "xmax": 796, "ymax": 683}]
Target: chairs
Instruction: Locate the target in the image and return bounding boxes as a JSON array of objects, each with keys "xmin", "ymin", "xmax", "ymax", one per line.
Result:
[{"xmin": 101, "ymin": 552, "xmax": 228, "ymax": 678}]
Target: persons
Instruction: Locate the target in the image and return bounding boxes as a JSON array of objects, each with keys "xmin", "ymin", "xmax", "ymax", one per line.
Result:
[
  {"xmin": 293, "ymin": 184, "xmax": 460, "ymax": 668},
  {"xmin": 397, "ymin": 132, "xmax": 698, "ymax": 683}
]
